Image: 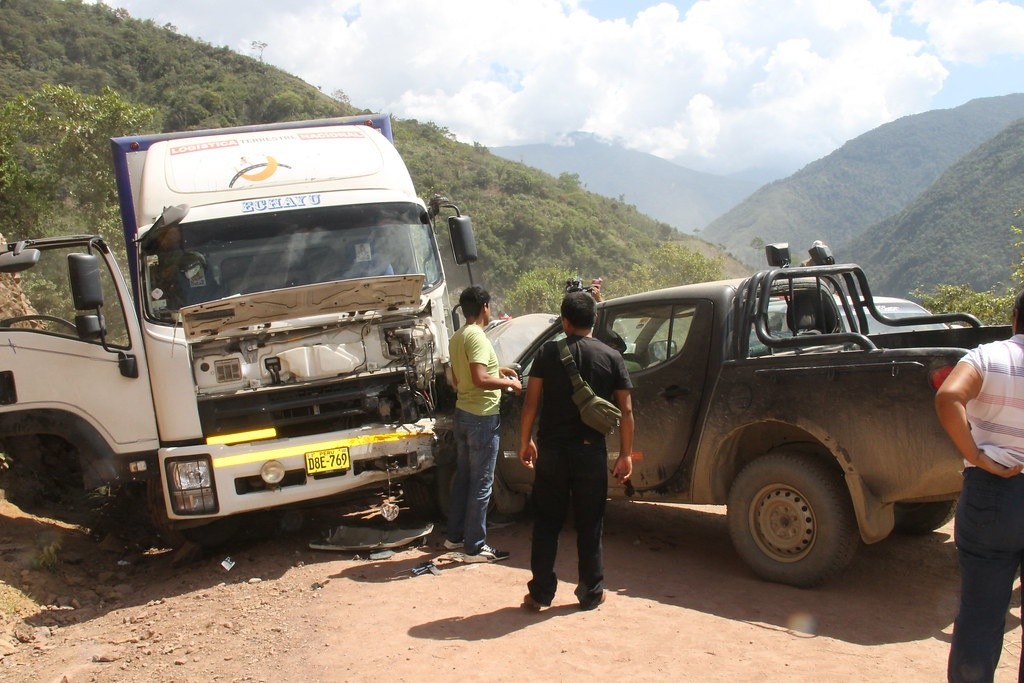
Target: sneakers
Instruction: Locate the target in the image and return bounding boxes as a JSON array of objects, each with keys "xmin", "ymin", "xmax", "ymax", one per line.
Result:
[
  {"xmin": 464, "ymin": 545, "xmax": 510, "ymax": 563},
  {"xmin": 444, "ymin": 540, "xmax": 466, "ymax": 549}
]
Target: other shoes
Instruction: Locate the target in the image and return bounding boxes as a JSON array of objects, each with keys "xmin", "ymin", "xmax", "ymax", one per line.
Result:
[
  {"xmin": 528, "ymin": 585, "xmax": 548, "ymax": 606},
  {"xmin": 582, "ymin": 592, "xmax": 607, "ymax": 610}
]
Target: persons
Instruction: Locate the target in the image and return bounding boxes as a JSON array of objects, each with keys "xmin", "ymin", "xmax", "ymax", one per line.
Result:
[
  {"xmin": 444, "ymin": 285, "xmax": 522, "ymax": 564},
  {"xmin": 518, "ymin": 279, "xmax": 635, "ymax": 610},
  {"xmin": 935, "ymin": 290, "xmax": 1024, "ymax": 683}
]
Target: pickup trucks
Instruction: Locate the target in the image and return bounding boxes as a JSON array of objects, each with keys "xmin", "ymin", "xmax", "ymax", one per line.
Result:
[{"xmin": 435, "ymin": 241, "xmax": 1017, "ymax": 593}]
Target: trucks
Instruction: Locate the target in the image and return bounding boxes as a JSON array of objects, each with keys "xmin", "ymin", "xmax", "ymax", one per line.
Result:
[{"xmin": 0, "ymin": 110, "xmax": 484, "ymax": 554}]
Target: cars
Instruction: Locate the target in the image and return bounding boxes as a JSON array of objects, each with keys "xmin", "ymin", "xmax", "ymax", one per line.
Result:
[{"xmin": 732, "ymin": 290, "xmax": 950, "ymax": 339}]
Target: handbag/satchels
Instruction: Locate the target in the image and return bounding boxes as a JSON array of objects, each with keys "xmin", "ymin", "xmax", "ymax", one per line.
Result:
[{"xmin": 557, "ymin": 338, "xmax": 622, "ymax": 435}]
